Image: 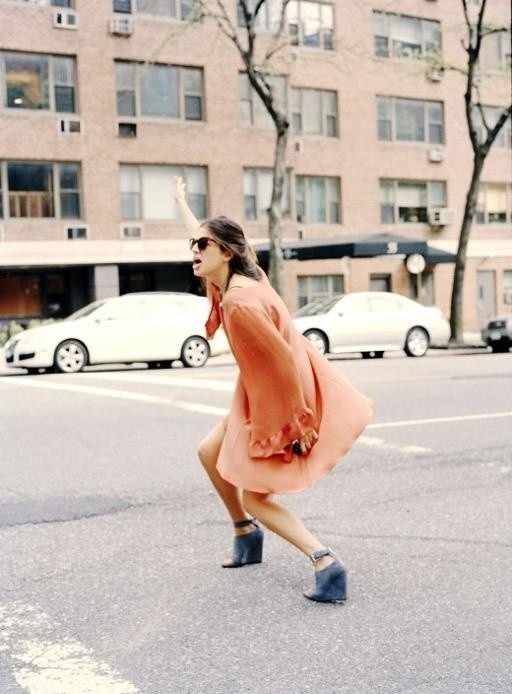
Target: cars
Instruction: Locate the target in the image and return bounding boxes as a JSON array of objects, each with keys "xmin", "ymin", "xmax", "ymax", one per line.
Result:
[
  {"xmin": 293, "ymin": 290, "xmax": 451, "ymax": 357},
  {"xmin": 479, "ymin": 317, "xmax": 512, "ymax": 353},
  {"xmin": 1, "ymin": 290, "xmax": 230, "ymax": 374}
]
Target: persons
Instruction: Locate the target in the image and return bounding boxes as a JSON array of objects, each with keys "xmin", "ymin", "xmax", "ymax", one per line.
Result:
[{"xmin": 175, "ymin": 176, "xmax": 374, "ymax": 601}]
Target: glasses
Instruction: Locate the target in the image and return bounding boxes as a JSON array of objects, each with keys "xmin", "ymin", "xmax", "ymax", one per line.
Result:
[{"xmin": 189, "ymin": 236, "xmax": 222, "ymax": 251}]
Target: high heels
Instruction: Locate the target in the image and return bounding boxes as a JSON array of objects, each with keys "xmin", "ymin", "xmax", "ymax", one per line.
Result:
[
  {"xmin": 221, "ymin": 519, "xmax": 263, "ymax": 568},
  {"xmin": 303, "ymin": 547, "xmax": 348, "ymax": 604}
]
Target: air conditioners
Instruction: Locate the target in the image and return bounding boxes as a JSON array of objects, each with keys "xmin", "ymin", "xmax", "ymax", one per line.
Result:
[
  {"xmin": 430, "ymin": 205, "xmax": 456, "ymax": 229},
  {"xmin": 58, "ymin": 117, "xmax": 81, "ymax": 137},
  {"xmin": 50, "ymin": 8, "xmax": 136, "ymax": 38},
  {"xmin": 63, "ymin": 222, "xmax": 144, "ymax": 243}
]
